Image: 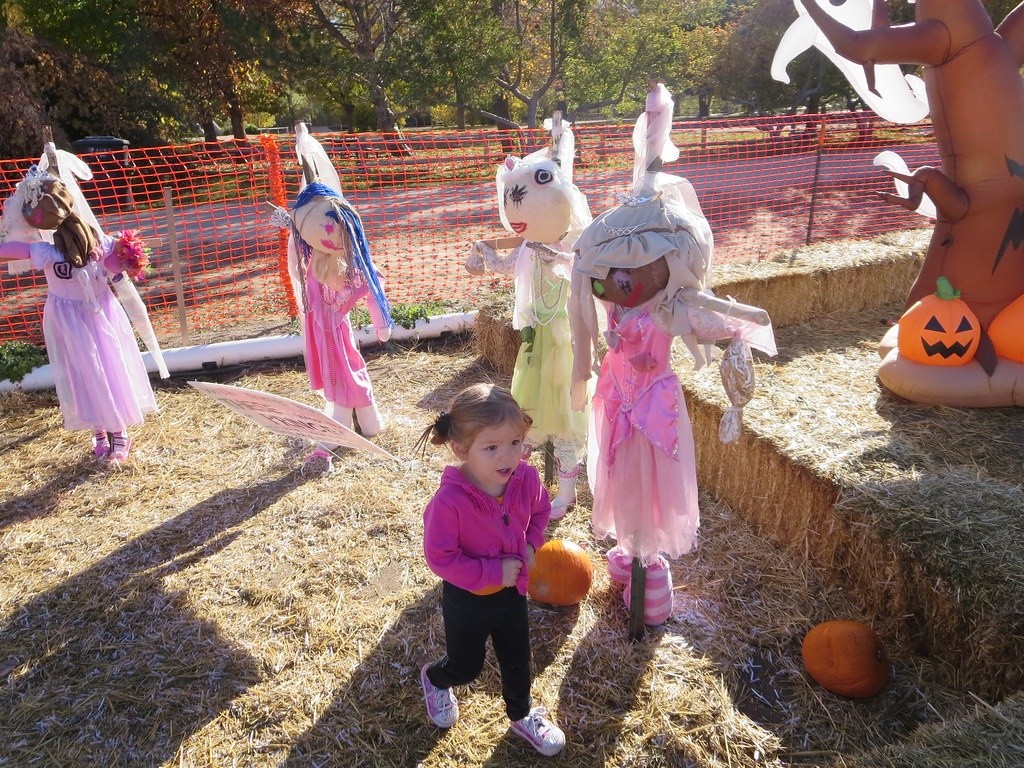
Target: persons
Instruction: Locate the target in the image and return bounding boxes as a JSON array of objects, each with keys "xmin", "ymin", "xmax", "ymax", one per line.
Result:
[{"xmin": 409, "ymin": 381, "xmax": 568, "ymax": 757}]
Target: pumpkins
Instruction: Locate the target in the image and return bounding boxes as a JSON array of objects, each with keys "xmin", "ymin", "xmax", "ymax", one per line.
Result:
[
  {"xmin": 801, "ymin": 621, "xmax": 888, "ymax": 698},
  {"xmin": 526, "ymin": 541, "xmax": 593, "ymax": 605}
]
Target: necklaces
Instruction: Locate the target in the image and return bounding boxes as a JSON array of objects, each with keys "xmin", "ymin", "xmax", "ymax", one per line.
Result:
[{"xmin": 531, "ymin": 252, "xmax": 565, "ymax": 327}]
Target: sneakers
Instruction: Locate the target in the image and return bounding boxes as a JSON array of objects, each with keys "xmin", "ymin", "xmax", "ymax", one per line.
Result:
[
  {"xmin": 510, "ymin": 707, "xmax": 566, "ymax": 755},
  {"xmin": 421, "ymin": 661, "xmax": 460, "ymax": 728}
]
